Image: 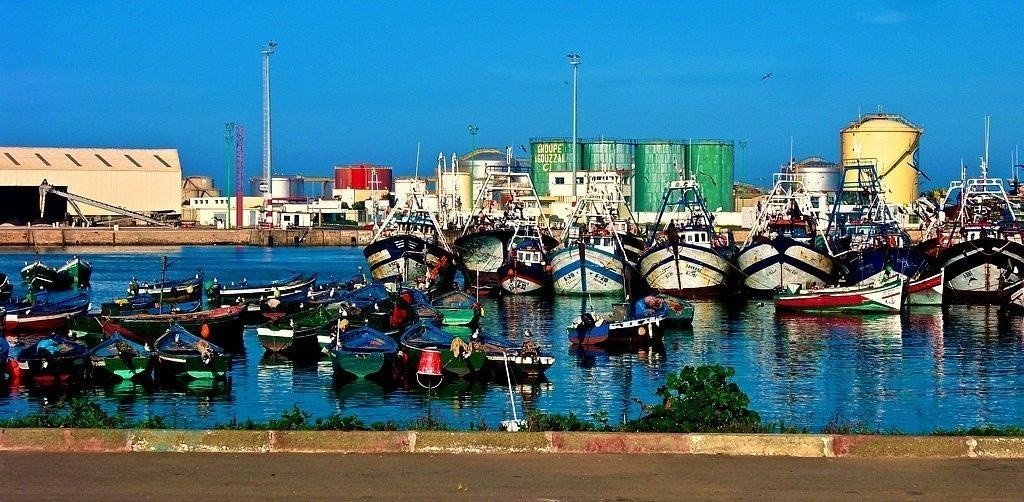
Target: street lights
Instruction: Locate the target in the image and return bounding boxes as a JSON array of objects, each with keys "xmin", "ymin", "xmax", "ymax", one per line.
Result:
[
  {"xmin": 568, "ymin": 49, "xmax": 583, "ymax": 213},
  {"xmin": 223, "ymin": 122, "xmax": 235, "ymax": 228},
  {"xmin": 468, "ymin": 124, "xmax": 479, "ymax": 177},
  {"xmin": 261, "ymin": 41, "xmax": 277, "ymax": 192}
]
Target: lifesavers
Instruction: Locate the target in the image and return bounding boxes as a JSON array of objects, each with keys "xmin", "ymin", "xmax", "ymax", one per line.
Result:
[
  {"xmin": 940, "ymin": 236, "xmax": 952, "ymax": 248},
  {"xmin": 712, "ymin": 235, "xmax": 727, "ymax": 246}
]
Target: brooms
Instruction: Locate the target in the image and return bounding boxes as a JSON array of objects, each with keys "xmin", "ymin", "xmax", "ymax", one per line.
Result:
[{"xmin": 501, "ymin": 351, "xmax": 528, "ymax": 433}]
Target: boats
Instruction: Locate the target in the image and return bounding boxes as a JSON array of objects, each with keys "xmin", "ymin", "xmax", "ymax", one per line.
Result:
[
  {"xmin": 637, "ymin": 165, "xmax": 729, "ymax": 292},
  {"xmin": 771, "ymin": 267, "xmax": 942, "ymax": 315},
  {"xmin": 827, "ymin": 156, "xmax": 932, "ymax": 283},
  {"xmin": 736, "ymin": 166, "xmax": 833, "ymax": 294},
  {"xmin": 566, "ymin": 290, "xmax": 695, "ymax": 353},
  {"xmin": 939, "ymin": 176, "xmax": 1024, "ymax": 310}
]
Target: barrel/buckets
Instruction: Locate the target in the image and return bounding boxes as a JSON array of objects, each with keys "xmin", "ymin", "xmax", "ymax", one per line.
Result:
[{"xmin": 415, "ymin": 351, "xmax": 445, "ymax": 389}]
